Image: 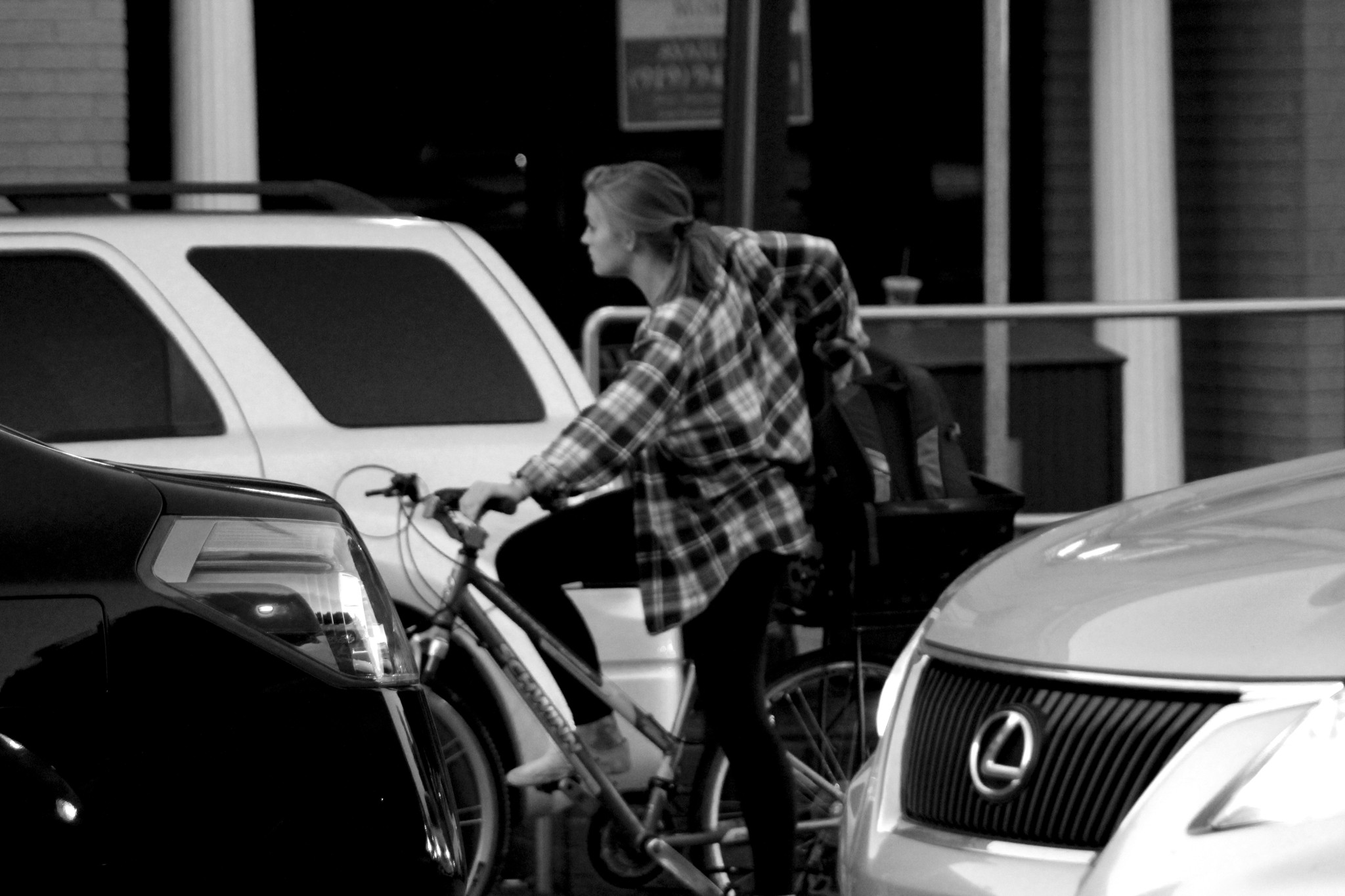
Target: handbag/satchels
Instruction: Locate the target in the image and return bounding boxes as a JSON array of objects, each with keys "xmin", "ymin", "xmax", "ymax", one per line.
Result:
[{"xmin": 799, "ymin": 342, "xmax": 875, "ymax": 504}]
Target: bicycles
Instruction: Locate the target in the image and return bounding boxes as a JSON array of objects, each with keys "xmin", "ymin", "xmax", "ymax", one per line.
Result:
[{"xmin": 364, "ymin": 470, "xmax": 896, "ymax": 896}]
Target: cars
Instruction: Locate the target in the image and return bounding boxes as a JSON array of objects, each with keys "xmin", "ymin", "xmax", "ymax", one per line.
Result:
[{"xmin": 1, "ymin": 420, "xmax": 473, "ymax": 896}]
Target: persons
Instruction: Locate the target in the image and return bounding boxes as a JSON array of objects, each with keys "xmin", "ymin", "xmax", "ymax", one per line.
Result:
[{"xmin": 459, "ymin": 161, "xmax": 871, "ymax": 896}]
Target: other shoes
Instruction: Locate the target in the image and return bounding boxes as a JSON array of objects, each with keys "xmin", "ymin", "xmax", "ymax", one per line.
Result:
[{"xmin": 505, "ymin": 738, "xmax": 632, "ymax": 786}]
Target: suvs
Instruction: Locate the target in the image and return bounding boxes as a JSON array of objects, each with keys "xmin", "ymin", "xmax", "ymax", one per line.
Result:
[{"xmin": 1, "ymin": 176, "xmax": 702, "ymax": 896}]
